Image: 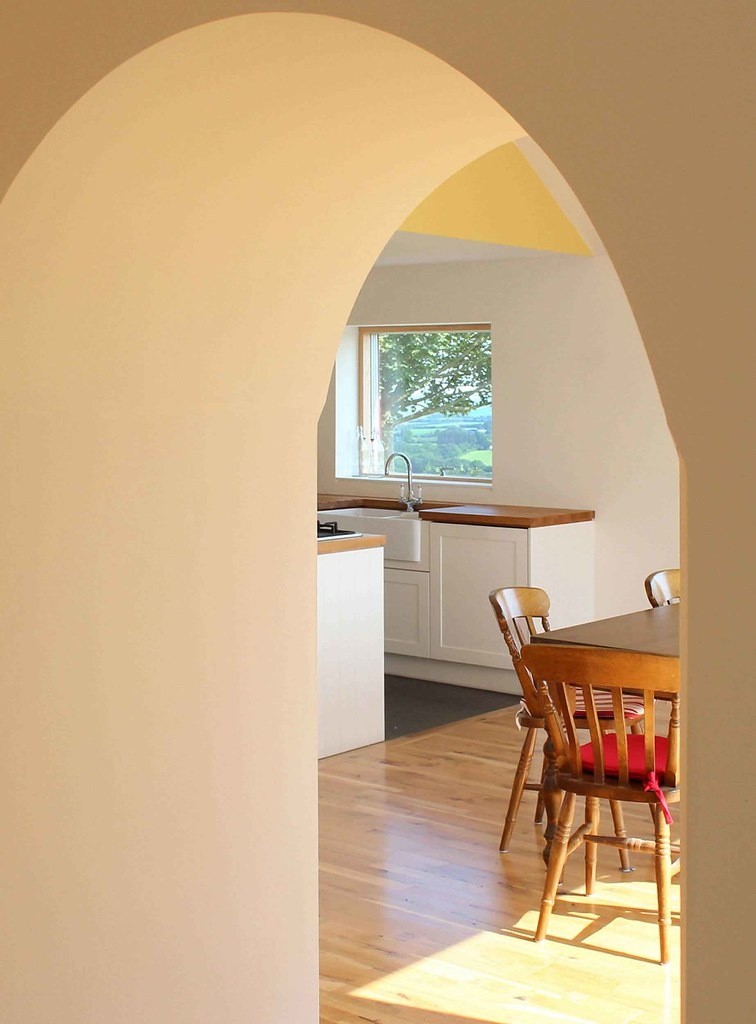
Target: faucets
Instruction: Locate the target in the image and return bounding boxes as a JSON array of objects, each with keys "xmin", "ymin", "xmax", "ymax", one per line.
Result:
[{"xmin": 384, "ymin": 452, "xmax": 415, "ymax": 500}]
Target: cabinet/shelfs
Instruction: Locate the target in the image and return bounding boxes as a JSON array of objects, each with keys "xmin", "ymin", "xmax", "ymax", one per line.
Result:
[{"xmin": 384, "ymin": 519, "xmax": 528, "ymax": 695}]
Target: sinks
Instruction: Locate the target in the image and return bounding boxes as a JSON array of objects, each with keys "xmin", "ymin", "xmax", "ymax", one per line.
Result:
[
  {"xmin": 317, "ymin": 508, "xmax": 402, "ymax": 560},
  {"xmin": 385, "ymin": 511, "xmax": 422, "ymax": 561}
]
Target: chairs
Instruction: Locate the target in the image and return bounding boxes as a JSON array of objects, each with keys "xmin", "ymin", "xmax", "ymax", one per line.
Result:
[
  {"xmin": 488, "ymin": 586, "xmax": 657, "ymax": 873},
  {"xmin": 521, "ymin": 644, "xmax": 681, "ymax": 963},
  {"xmin": 645, "ymin": 569, "xmax": 681, "ymax": 608}
]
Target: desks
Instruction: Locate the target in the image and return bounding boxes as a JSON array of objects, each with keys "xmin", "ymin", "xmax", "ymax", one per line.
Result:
[{"xmin": 529, "ymin": 603, "xmax": 680, "ymax": 885}]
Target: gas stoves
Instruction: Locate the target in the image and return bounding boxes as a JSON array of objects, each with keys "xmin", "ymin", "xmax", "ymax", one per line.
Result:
[{"xmin": 317, "ymin": 519, "xmax": 362, "ymax": 542}]
